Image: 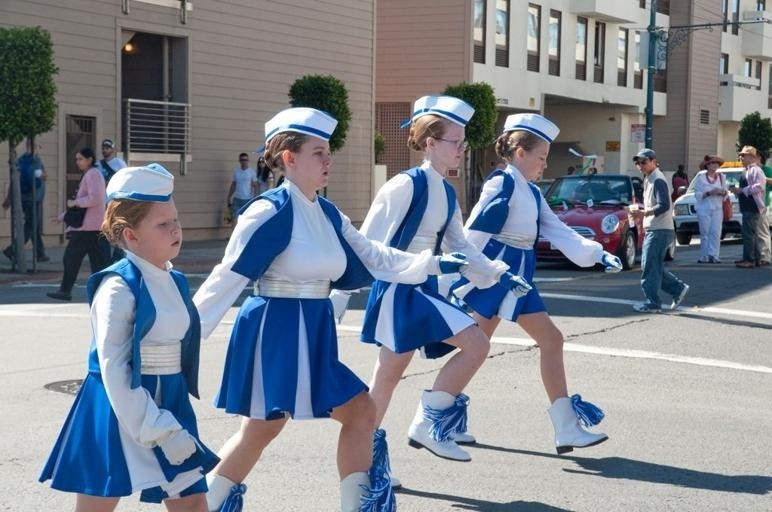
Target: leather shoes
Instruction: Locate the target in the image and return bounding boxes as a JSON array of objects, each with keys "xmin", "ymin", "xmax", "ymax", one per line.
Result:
[{"xmin": 735, "ymin": 257, "xmax": 754, "ymax": 269}]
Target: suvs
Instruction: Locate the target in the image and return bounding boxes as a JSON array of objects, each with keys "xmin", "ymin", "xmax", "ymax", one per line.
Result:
[{"xmin": 673, "ymin": 168, "xmax": 772, "ymax": 245}]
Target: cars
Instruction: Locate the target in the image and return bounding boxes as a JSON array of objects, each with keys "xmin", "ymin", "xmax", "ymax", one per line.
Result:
[{"xmin": 535, "ymin": 175, "xmax": 676, "ymax": 268}]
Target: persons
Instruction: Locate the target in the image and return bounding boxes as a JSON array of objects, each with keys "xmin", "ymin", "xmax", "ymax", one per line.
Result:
[
  {"xmin": 690, "ymin": 155, "xmax": 728, "ymax": 264},
  {"xmin": 228, "ymin": 153, "xmax": 258, "ymax": 230},
  {"xmin": 2, "ymin": 137, "xmax": 49, "ymax": 264},
  {"xmin": 727, "ymin": 145, "xmax": 766, "ymax": 267},
  {"xmin": 46, "ymin": 149, "xmax": 111, "ymax": 300},
  {"xmin": 628, "ymin": 148, "xmax": 689, "ymax": 312},
  {"xmin": 438, "ymin": 114, "xmax": 622, "ymax": 455},
  {"xmin": 672, "ymin": 165, "xmax": 688, "ymax": 197},
  {"xmin": 191, "ymin": 107, "xmax": 468, "ymax": 512},
  {"xmin": 40, "ymin": 164, "xmax": 221, "ymax": 512},
  {"xmin": 754, "ymin": 151, "xmax": 772, "ymax": 266},
  {"xmin": 95, "ymin": 140, "xmax": 127, "ymax": 183},
  {"xmin": 329, "ymin": 96, "xmax": 533, "ymax": 490},
  {"xmin": 257, "ymin": 156, "xmax": 274, "ymax": 195}
]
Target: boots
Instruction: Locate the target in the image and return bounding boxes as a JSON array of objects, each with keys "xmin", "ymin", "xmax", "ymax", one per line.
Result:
[
  {"xmin": 199, "ymin": 464, "xmax": 247, "ymax": 512},
  {"xmin": 544, "ymin": 392, "xmax": 610, "ymax": 455},
  {"xmin": 449, "ymin": 398, "xmax": 478, "ymax": 446},
  {"xmin": 406, "ymin": 384, "xmax": 478, "ymax": 465},
  {"xmin": 338, "ymin": 471, "xmax": 378, "ymax": 512}
]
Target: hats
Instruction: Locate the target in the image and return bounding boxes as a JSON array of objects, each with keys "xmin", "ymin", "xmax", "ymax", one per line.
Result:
[
  {"xmin": 699, "ymin": 154, "xmax": 724, "ymax": 170},
  {"xmin": 101, "ymin": 164, "xmax": 176, "ymax": 206},
  {"xmin": 503, "ymin": 110, "xmax": 561, "ymax": 145},
  {"xmin": 399, "ymin": 94, "xmax": 476, "ymax": 129},
  {"xmin": 101, "ymin": 138, "xmax": 115, "ymax": 149},
  {"xmin": 255, "ymin": 106, "xmax": 339, "ymax": 153},
  {"xmin": 632, "ymin": 147, "xmax": 656, "ymax": 163},
  {"xmin": 737, "ymin": 145, "xmax": 757, "ymax": 158}
]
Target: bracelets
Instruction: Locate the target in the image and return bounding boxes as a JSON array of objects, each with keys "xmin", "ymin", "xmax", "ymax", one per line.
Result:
[{"xmin": 707, "ymin": 191, "xmax": 709, "ymax": 196}]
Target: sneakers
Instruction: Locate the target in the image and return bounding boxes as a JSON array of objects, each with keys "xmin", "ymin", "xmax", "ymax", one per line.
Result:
[
  {"xmin": 36, "ymin": 255, "xmax": 51, "ymax": 263},
  {"xmin": 712, "ymin": 255, "xmax": 722, "ymax": 264},
  {"xmin": 669, "ymin": 280, "xmax": 689, "ymax": 309},
  {"xmin": 631, "ymin": 302, "xmax": 661, "ymax": 315},
  {"xmin": 45, "ymin": 288, "xmax": 71, "ymax": 302},
  {"xmin": 698, "ymin": 256, "xmax": 710, "ymax": 264},
  {"xmin": 3, "ymin": 246, "xmax": 14, "ymax": 262}
]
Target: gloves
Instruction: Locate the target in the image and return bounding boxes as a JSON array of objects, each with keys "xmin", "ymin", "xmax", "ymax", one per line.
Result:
[
  {"xmin": 438, "ymin": 250, "xmax": 470, "ymax": 274},
  {"xmin": 499, "ymin": 272, "xmax": 533, "ymax": 299},
  {"xmin": 598, "ymin": 250, "xmax": 623, "ymax": 276},
  {"xmin": 159, "ymin": 428, "xmax": 197, "ymax": 466}
]
